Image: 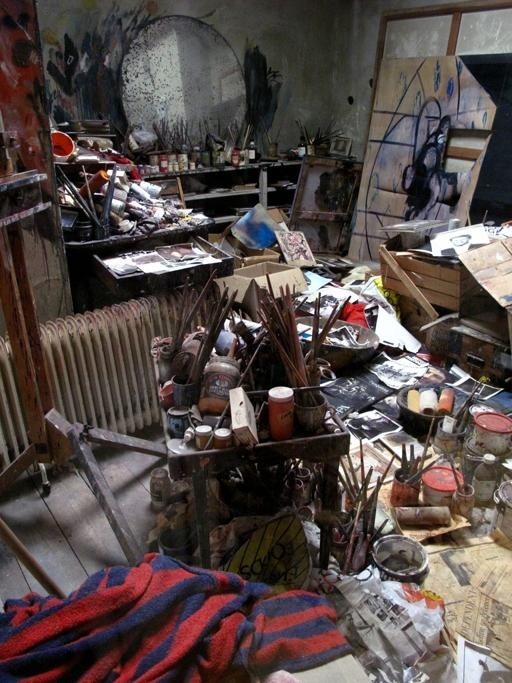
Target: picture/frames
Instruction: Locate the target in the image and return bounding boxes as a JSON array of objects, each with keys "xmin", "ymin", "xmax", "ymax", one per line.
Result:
[{"xmin": 328, "ymin": 134, "xmax": 353, "ymax": 157}]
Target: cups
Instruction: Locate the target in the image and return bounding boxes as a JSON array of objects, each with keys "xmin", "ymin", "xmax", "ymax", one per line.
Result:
[
  {"xmin": 283, "ymin": 467, "xmax": 311, "ymax": 507},
  {"xmin": 93, "ymin": 225, "xmax": 109, "ymax": 239},
  {"xmin": 50, "ymin": 130, "xmax": 75, "ymax": 162},
  {"xmin": 328, "ymin": 512, "xmax": 354, "ymax": 566},
  {"xmin": 390, "ymin": 467, "xmax": 420, "ymax": 509},
  {"xmin": 100, "ymin": 180, "xmax": 162, "ymax": 215},
  {"xmin": 305, "ymin": 144, "xmax": 315, "ymax": 156},
  {"xmin": 295, "ymin": 392, "xmax": 336, "ymax": 435},
  {"xmin": 158, "ymin": 528, "xmax": 197, "ymax": 565},
  {"xmin": 172, "ymin": 373, "xmax": 194, "ymax": 405},
  {"xmin": 268, "ymin": 143, "xmax": 279, "ymax": 157}
]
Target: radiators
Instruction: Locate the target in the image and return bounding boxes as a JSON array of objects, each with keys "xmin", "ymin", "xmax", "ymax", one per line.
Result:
[{"xmin": 1, "ymin": 285, "xmax": 220, "ymax": 484}]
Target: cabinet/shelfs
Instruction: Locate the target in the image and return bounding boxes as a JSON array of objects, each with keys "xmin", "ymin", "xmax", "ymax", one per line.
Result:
[
  {"xmin": 59, "ymin": 215, "xmax": 218, "ymax": 309},
  {"xmin": 144, "ymin": 152, "xmax": 307, "ymax": 228}
]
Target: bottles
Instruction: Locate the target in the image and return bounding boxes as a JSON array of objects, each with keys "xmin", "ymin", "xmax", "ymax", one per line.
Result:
[
  {"xmin": 149, "ymin": 467, "xmax": 172, "ymax": 508},
  {"xmin": 147, "ymin": 141, "xmax": 257, "ymax": 173},
  {"xmin": 450, "ymin": 485, "xmax": 474, "ymax": 521},
  {"xmin": 157, "ymin": 345, "xmax": 173, "ymax": 381},
  {"xmin": 297, "ymin": 137, "xmax": 307, "ymax": 159},
  {"xmin": 74, "ymin": 219, "xmax": 92, "ymax": 241},
  {"xmin": 198, "ymin": 356, "xmax": 241, "ymax": 416},
  {"xmin": 473, "ymin": 452, "xmax": 497, "ymax": 508}
]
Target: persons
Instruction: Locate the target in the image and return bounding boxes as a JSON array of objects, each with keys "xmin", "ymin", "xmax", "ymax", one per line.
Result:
[
  {"xmin": 441, "ymin": 234, "xmax": 487, "ymax": 257},
  {"xmin": 419, "ymin": 366, "xmax": 455, "ymax": 385},
  {"xmin": 300, "ymin": 295, "xmax": 339, "ymax": 315}
]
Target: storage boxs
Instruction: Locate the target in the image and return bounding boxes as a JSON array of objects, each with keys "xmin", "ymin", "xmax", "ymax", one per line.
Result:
[
  {"xmin": 455, "ymin": 316, "xmax": 510, "ymax": 389},
  {"xmin": 395, "ymin": 296, "xmax": 455, "ymax": 335},
  {"xmin": 207, "ymin": 232, "xmax": 284, "ymax": 268},
  {"xmin": 215, "ymin": 259, "xmax": 310, "ymax": 317},
  {"xmin": 376, "ymin": 244, "xmax": 477, "ymax": 314}
]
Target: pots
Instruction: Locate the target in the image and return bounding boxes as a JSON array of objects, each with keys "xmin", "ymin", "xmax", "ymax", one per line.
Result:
[{"xmin": 294, "ymin": 316, "xmax": 380, "ymax": 375}]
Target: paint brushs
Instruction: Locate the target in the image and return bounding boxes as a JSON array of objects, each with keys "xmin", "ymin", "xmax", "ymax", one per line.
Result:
[
  {"xmin": 58, "ymin": 164, "xmax": 102, "ymax": 227},
  {"xmin": 153, "ymin": 117, "xmax": 270, "ymax": 151},
  {"xmin": 296, "ymin": 117, "xmax": 346, "ymax": 144},
  {"xmin": 169, "ymin": 268, "xmax": 351, "ymax": 423},
  {"xmin": 449, "ymin": 381, "xmax": 487, "ymax": 492},
  {"xmin": 338, "ymin": 417, "xmax": 445, "ymax": 574}
]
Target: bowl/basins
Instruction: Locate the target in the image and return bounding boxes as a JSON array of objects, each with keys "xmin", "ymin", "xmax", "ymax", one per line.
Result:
[
  {"xmin": 397, "ymin": 385, "xmax": 465, "ymax": 426},
  {"xmin": 80, "ymin": 119, "xmax": 110, "ymax": 133},
  {"xmin": 372, "ymin": 534, "xmax": 428, "ymax": 578}
]
